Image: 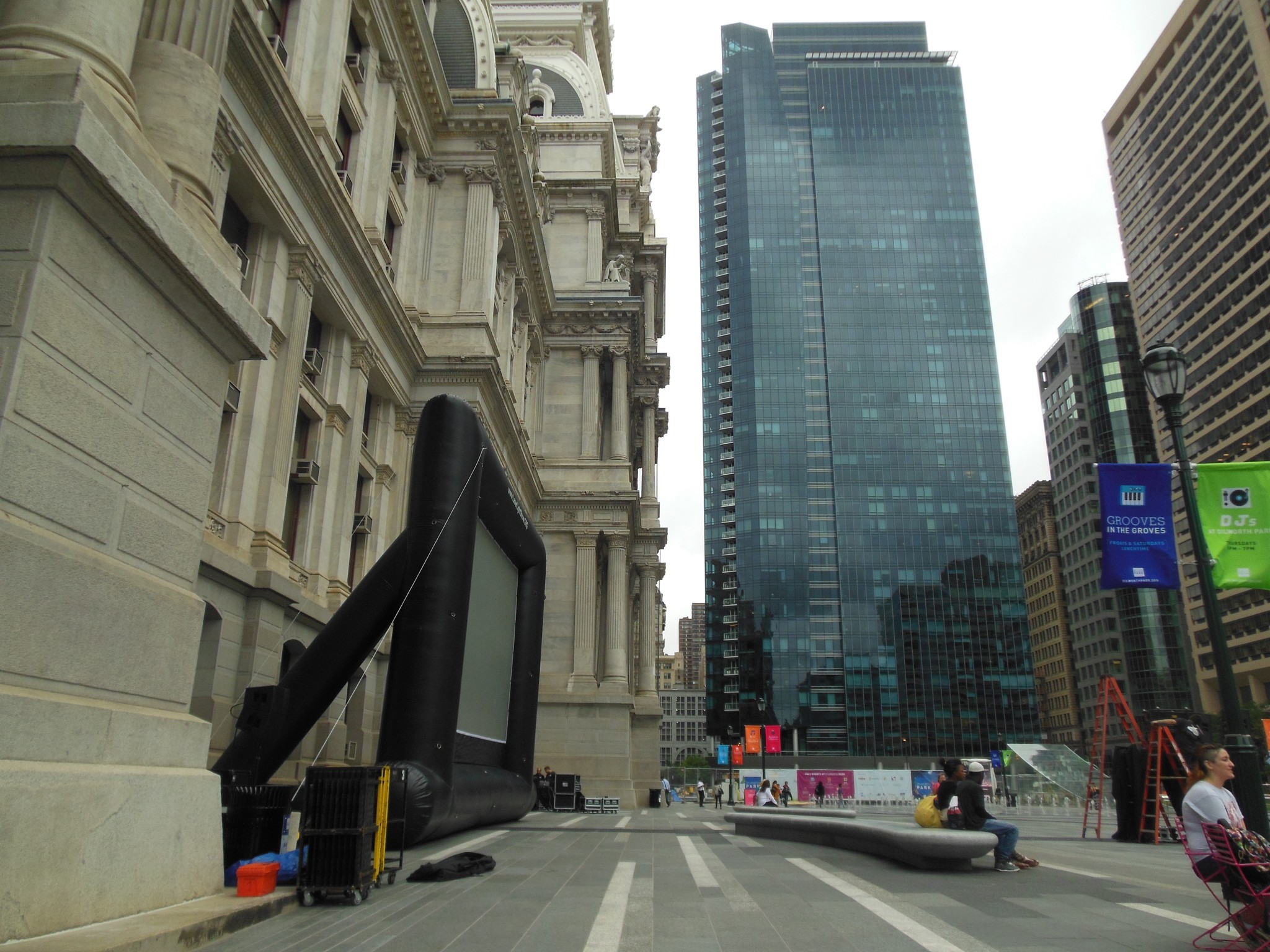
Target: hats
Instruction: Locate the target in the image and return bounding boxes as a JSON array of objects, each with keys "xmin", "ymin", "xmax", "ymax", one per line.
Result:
[{"xmin": 968, "ymin": 762, "xmax": 988, "ymax": 772}]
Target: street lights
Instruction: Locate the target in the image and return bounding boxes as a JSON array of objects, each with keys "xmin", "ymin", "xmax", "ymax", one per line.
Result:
[
  {"xmin": 997, "ymin": 733, "xmax": 1010, "ymax": 808},
  {"xmin": 757, "ymin": 698, "xmax": 768, "ymax": 780},
  {"xmin": 1138, "ymin": 336, "xmax": 1270, "ymax": 842},
  {"xmin": 727, "ymin": 724, "xmax": 736, "ymax": 806}
]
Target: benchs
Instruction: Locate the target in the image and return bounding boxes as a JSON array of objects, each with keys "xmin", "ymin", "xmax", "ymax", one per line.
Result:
[{"xmin": 724, "ymin": 805, "xmax": 999, "ymax": 870}]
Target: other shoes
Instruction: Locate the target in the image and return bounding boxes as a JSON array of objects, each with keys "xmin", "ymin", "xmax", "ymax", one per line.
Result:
[
  {"xmin": 1013, "ymin": 850, "xmax": 1039, "ymax": 867},
  {"xmin": 1009, "ymin": 858, "xmax": 1030, "ymax": 869},
  {"xmin": 994, "ymin": 860, "xmax": 1020, "ymax": 872}
]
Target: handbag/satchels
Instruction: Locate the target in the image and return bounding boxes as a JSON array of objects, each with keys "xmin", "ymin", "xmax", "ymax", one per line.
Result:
[
  {"xmin": 914, "ymin": 795, "xmax": 943, "ymax": 828},
  {"xmin": 718, "ymin": 788, "xmax": 724, "ymax": 794},
  {"xmin": 666, "ymin": 789, "xmax": 669, "ymax": 793},
  {"xmin": 744, "ymin": 789, "xmax": 756, "ymax": 805}
]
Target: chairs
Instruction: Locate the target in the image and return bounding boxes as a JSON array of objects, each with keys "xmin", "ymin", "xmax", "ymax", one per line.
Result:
[{"xmin": 1175, "ymin": 816, "xmax": 1270, "ymax": 952}]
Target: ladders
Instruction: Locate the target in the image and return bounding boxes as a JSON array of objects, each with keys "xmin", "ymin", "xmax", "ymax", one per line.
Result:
[
  {"xmin": 1082, "ymin": 674, "xmax": 1178, "ymax": 840},
  {"xmin": 1138, "ymin": 726, "xmax": 1192, "ymax": 845}
]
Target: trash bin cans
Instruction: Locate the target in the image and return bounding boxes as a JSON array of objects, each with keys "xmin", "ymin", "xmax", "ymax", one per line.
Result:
[
  {"xmin": 220, "ymin": 783, "xmax": 297, "ymax": 868},
  {"xmin": 649, "ymin": 789, "xmax": 662, "ymax": 808},
  {"xmin": 298, "ymin": 765, "xmax": 391, "ymax": 906}
]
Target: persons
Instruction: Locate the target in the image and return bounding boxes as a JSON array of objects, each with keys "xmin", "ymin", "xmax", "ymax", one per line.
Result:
[
  {"xmin": 1086, "ymin": 785, "xmax": 1101, "ymax": 810},
  {"xmin": 1180, "ymin": 743, "xmax": 1270, "ymax": 935},
  {"xmin": 756, "ymin": 780, "xmax": 784, "ymax": 807},
  {"xmin": 697, "ymin": 777, "xmax": 705, "ymax": 807},
  {"xmin": 954, "ymin": 762, "xmax": 1021, "ymax": 872},
  {"xmin": 814, "ymin": 781, "xmax": 825, "ymax": 808},
  {"xmin": 713, "ymin": 780, "xmax": 723, "ymax": 809},
  {"xmin": 661, "ymin": 776, "xmax": 670, "ymax": 807},
  {"xmin": 782, "ymin": 781, "xmax": 791, "ymax": 808},
  {"xmin": 931, "ymin": 757, "xmax": 1039, "ymax": 869},
  {"xmin": 837, "ymin": 783, "xmax": 846, "ymax": 808},
  {"xmin": 991, "ymin": 785, "xmax": 1002, "ymax": 807},
  {"xmin": 532, "ymin": 766, "xmax": 558, "ymax": 812}
]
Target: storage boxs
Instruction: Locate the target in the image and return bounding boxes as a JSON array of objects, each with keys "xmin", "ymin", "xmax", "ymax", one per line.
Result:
[
  {"xmin": 552, "ymin": 774, "xmax": 620, "ymax": 814},
  {"xmin": 237, "ymin": 863, "xmax": 280, "ymax": 897}
]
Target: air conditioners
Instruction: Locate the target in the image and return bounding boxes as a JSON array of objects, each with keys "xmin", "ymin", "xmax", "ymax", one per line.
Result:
[
  {"xmin": 223, "ymin": 379, "xmax": 241, "ymax": 413},
  {"xmin": 391, "ymin": 161, "xmax": 406, "ymax": 185},
  {"xmin": 345, "ymin": 53, "xmax": 365, "ymax": 83},
  {"xmin": 266, "ymin": 35, "xmax": 289, "ymax": 72},
  {"xmin": 335, "ymin": 170, "xmax": 353, "ymax": 199},
  {"xmin": 305, "ymin": 348, "xmax": 324, "ymax": 375},
  {"xmin": 352, "ymin": 513, "xmax": 373, "ymax": 534},
  {"xmin": 229, "ymin": 243, "xmax": 250, "ymax": 280},
  {"xmin": 386, "ymin": 265, "xmax": 395, "ymax": 284},
  {"xmin": 290, "ymin": 458, "xmax": 320, "ymax": 485}
]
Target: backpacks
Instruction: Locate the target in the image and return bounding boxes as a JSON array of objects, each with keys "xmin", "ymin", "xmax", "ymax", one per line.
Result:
[
  {"xmin": 947, "ymin": 795, "xmax": 967, "ymax": 829},
  {"xmin": 1206, "ymin": 818, "xmax": 1270, "ymax": 904}
]
